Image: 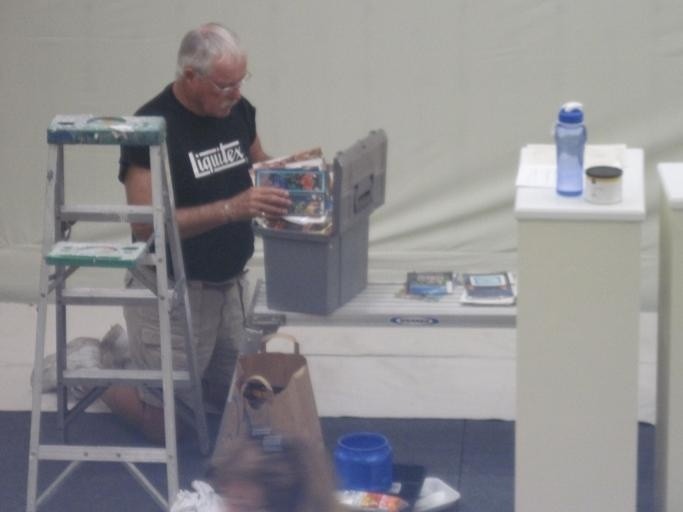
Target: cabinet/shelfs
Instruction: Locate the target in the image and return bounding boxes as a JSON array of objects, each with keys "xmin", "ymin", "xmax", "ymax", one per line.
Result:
[{"xmin": 228, "ymin": 277, "xmax": 658, "ymax": 429}]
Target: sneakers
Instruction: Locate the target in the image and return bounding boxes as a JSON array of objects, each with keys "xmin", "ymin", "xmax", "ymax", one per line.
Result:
[{"xmin": 31, "ymin": 324, "xmax": 134, "ymax": 401}]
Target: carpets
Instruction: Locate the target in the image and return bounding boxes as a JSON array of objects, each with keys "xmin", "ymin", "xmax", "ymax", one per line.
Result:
[{"xmin": 1, "ymin": 411, "xmax": 655, "ymax": 511}]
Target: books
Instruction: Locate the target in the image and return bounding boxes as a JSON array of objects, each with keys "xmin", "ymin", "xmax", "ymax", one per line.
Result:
[{"xmin": 395, "ymin": 270, "xmax": 515, "ymax": 306}]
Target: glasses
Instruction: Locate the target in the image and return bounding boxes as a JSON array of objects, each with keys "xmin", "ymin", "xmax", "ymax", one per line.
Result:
[{"xmin": 198, "ymin": 69, "xmax": 254, "ymax": 96}]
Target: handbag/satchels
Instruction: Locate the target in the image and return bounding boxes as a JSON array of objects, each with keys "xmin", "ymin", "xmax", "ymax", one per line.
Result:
[{"xmin": 208, "ymin": 333, "xmax": 336, "ymax": 512}]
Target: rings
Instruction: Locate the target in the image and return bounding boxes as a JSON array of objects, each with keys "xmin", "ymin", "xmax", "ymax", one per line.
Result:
[{"xmin": 262, "ymin": 210, "xmax": 267, "ymax": 219}]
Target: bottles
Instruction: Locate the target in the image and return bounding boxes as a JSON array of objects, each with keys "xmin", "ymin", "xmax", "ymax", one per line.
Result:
[
  {"xmin": 331, "ymin": 432, "xmax": 393, "ymax": 494},
  {"xmin": 553, "ymin": 101, "xmax": 588, "ymax": 196}
]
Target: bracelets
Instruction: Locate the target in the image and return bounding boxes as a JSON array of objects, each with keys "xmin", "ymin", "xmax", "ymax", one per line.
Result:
[{"xmin": 222, "ymin": 197, "xmax": 236, "ymax": 223}]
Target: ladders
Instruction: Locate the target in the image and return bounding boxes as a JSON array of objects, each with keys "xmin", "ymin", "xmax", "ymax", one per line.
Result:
[{"xmin": 27, "ymin": 115, "xmax": 211, "ymax": 512}]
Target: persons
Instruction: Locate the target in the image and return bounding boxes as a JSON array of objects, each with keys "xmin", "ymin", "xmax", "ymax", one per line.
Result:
[
  {"xmin": 201, "ymin": 444, "xmax": 308, "ymax": 510},
  {"xmin": 31, "ymin": 23, "xmax": 292, "ymax": 444}
]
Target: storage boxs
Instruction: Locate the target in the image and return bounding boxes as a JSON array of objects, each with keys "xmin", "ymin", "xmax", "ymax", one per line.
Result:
[{"xmin": 253, "ymin": 129, "xmax": 388, "ymax": 316}]
